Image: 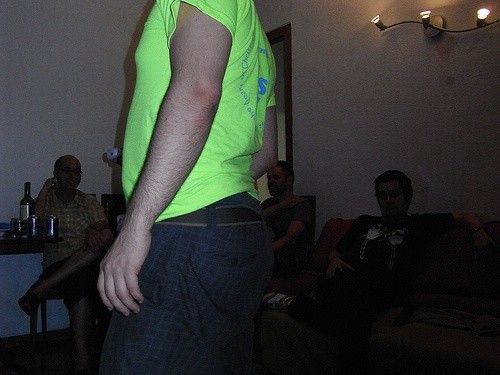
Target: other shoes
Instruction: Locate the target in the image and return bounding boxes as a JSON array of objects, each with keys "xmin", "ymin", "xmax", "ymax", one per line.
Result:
[
  {"xmin": 17, "ymin": 284, "xmax": 41, "ymax": 314},
  {"xmin": 263, "ymin": 291, "xmax": 295, "ymax": 313}
]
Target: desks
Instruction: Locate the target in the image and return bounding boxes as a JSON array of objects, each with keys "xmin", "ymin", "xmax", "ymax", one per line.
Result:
[{"xmin": 1, "ymin": 229, "xmax": 64, "ymax": 374}]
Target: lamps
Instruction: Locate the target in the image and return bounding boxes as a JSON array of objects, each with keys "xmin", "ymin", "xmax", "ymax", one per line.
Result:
[{"xmin": 372, "ymin": 4, "xmax": 498, "ymax": 38}]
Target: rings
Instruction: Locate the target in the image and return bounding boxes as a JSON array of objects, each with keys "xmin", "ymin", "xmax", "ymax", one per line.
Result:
[{"xmin": 95, "ymin": 238, "xmax": 100, "ymax": 241}]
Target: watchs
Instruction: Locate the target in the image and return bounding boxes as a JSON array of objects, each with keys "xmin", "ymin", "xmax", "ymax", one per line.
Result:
[{"xmin": 471, "ymin": 222, "xmax": 485, "ymax": 234}]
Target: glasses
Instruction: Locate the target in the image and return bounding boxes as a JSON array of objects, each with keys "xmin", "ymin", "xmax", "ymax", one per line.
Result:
[
  {"xmin": 374, "ymin": 188, "xmax": 404, "ymax": 200},
  {"xmin": 57, "ymin": 168, "xmax": 82, "ymax": 176}
]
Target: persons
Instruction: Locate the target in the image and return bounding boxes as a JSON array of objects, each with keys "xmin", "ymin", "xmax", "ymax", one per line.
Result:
[
  {"xmin": 96, "ymin": 0, "xmax": 279, "ymax": 375},
  {"xmin": 18, "ymin": 155, "xmax": 115, "ymax": 375},
  {"xmin": 258, "ymin": 161, "xmax": 497, "ymax": 375}
]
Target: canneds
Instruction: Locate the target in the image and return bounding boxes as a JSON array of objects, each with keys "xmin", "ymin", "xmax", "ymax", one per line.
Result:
[
  {"xmin": 46, "ymin": 215, "xmax": 59, "ymax": 237},
  {"xmin": 29, "ymin": 215, "xmax": 40, "ymax": 236}
]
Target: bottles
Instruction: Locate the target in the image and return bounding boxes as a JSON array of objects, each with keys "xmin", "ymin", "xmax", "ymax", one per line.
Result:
[{"xmin": 20, "ymin": 181, "xmax": 34, "ymax": 234}]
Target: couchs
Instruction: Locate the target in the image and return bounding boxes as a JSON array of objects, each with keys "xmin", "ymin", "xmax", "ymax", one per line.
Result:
[{"xmin": 261, "ymin": 218, "xmax": 499, "ymax": 374}]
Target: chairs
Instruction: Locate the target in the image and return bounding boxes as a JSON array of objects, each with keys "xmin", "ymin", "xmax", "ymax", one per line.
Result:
[
  {"xmin": 301, "ymin": 195, "xmax": 316, "ymax": 273},
  {"xmin": 29, "ymin": 194, "xmax": 124, "ymax": 365}
]
